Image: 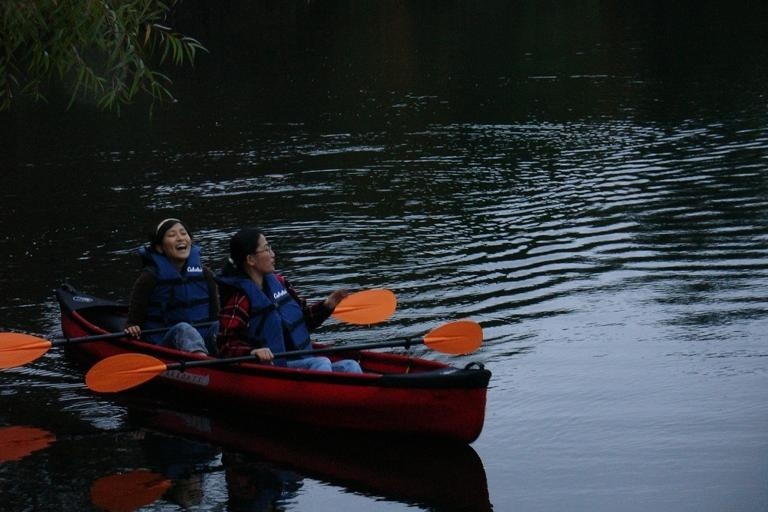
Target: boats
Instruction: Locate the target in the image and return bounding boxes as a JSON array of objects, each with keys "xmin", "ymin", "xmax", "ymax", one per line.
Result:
[
  {"xmin": 119, "ymin": 395, "xmax": 498, "ymax": 511},
  {"xmin": 57, "ymin": 278, "xmax": 493, "ymax": 453}
]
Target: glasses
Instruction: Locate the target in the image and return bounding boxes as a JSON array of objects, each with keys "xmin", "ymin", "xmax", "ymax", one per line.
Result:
[{"xmin": 254, "ymin": 244, "xmax": 272, "ymax": 255}]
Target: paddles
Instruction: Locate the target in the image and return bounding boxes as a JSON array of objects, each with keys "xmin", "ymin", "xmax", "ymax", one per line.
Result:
[
  {"xmin": 91, "ymin": 465, "xmax": 223, "ymax": 511},
  {"xmin": 0, "ymin": 289, "xmax": 397, "ymax": 370},
  {"xmin": 86, "ymin": 321, "xmax": 483, "ymax": 392},
  {"xmin": 0, "ymin": 425, "xmax": 59, "ymax": 464}
]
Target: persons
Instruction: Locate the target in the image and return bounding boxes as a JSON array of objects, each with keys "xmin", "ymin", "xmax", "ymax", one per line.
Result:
[
  {"xmin": 216, "ymin": 227, "xmax": 364, "ymax": 373},
  {"xmin": 123, "ymin": 217, "xmax": 219, "ymax": 357}
]
